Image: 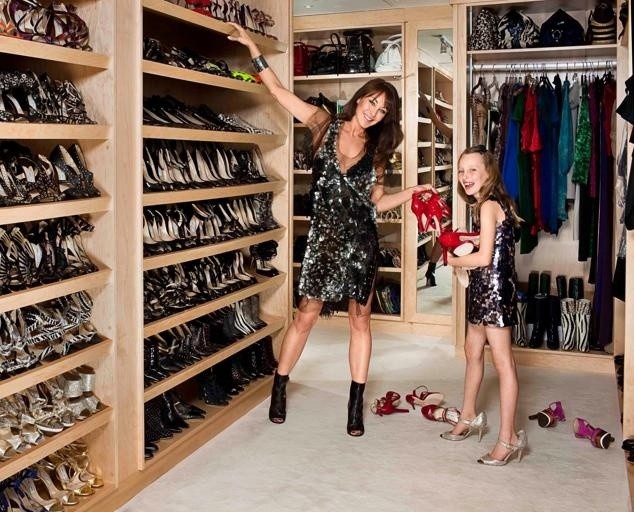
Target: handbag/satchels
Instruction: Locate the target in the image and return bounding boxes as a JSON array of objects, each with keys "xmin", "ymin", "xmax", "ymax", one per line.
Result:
[
  {"xmin": 293, "ymin": 28, "xmax": 403, "ymax": 78},
  {"xmin": 466, "ymin": 1, "xmax": 616, "ymax": 52}
]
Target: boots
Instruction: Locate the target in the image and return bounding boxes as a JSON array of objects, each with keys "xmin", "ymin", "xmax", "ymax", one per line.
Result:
[
  {"xmin": 346, "ymin": 379, "xmax": 368, "ymax": 438},
  {"xmin": 144, "ymin": 295, "xmax": 267, "ymax": 387},
  {"xmin": 424, "ymin": 262, "xmax": 439, "ymax": 286},
  {"xmin": 267, "ymin": 369, "xmax": 290, "ymax": 426},
  {"xmin": 145, "ymin": 336, "xmax": 279, "ymax": 455},
  {"xmin": 513, "ymin": 270, "xmax": 593, "ymax": 354}
]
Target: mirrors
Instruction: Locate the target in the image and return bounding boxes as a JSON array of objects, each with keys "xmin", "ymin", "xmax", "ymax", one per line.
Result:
[{"xmin": 416, "ymin": 26, "xmax": 455, "ymax": 320}]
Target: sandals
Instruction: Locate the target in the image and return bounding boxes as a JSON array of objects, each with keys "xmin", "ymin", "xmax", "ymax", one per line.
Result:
[
  {"xmin": 418, "ymin": 404, "xmax": 463, "ymax": 428},
  {"xmin": 0, "ymin": 0, "xmax": 91, "ymax": 49}
]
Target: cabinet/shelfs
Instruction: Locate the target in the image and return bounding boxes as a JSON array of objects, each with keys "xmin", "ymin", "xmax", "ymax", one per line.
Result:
[
  {"xmin": 293, "ymin": 7, "xmax": 406, "ymax": 326},
  {"xmin": 454, "ymin": 0, "xmax": 617, "ymax": 376},
  {"xmin": 417, "ymin": 59, "xmax": 454, "ymax": 284},
  {"xmin": 128, "ymin": 0, "xmax": 294, "ymax": 500},
  {"xmin": 0, "ymin": 0, "xmax": 128, "ymax": 512},
  {"xmin": 614, "ymin": 1, "xmax": 633, "ymax": 439}
]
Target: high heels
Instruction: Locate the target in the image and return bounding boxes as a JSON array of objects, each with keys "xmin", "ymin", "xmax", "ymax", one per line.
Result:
[
  {"xmin": 0, "ymin": 215, "xmax": 99, "ymax": 295},
  {"xmin": 438, "ymin": 410, "xmax": 488, "ymax": 444},
  {"xmin": 1, "ymin": 291, "xmax": 102, "ymax": 377},
  {"xmin": 0, "ymin": 144, "xmax": 101, "ymax": 202},
  {"xmin": 410, "ymin": 189, "xmax": 484, "ymax": 268},
  {"xmin": 572, "ymin": 416, "xmax": 617, "ymax": 453},
  {"xmin": 526, "ymin": 399, "xmax": 566, "ymax": 431},
  {"xmin": 405, "ymin": 385, "xmax": 446, "ymax": 412},
  {"xmin": 174, "ymin": 0, "xmax": 277, "ymax": 39},
  {"xmin": 144, "ymin": 240, "xmax": 279, "ymax": 322},
  {"xmin": 0, "ymin": 366, "xmax": 101, "ymax": 459},
  {"xmin": 369, "ymin": 389, "xmax": 410, "ymax": 419},
  {"xmin": 0, "ymin": 72, "xmax": 96, "ymax": 124},
  {"xmin": 478, "ymin": 428, "xmax": 529, "ymax": 468},
  {"xmin": 0, "ymin": 441, "xmax": 104, "ymax": 512}
]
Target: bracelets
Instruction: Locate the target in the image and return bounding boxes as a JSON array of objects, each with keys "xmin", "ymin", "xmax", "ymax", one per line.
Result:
[{"xmin": 251, "ymin": 55, "xmax": 269, "ymax": 74}]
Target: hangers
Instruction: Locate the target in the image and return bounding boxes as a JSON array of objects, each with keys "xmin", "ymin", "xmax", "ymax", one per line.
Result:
[{"xmin": 471, "ymin": 58, "xmax": 614, "ymax": 86}]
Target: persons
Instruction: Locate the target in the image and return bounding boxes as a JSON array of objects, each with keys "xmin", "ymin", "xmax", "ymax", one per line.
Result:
[
  {"xmin": 440, "ymin": 144, "xmax": 519, "ymax": 467},
  {"xmin": 228, "ymin": 21, "xmax": 438, "ymax": 437},
  {"xmin": 418, "ymin": 89, "xmax": 453, "ymax": 286}
]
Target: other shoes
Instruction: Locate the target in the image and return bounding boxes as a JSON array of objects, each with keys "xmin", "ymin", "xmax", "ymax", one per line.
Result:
[
  {"xmin": 143, "ymin": 193, "xmax": 279, "ymax": 254},
  {"xmin": 145, "ymin": 37, "xmax": 258, "ymax": 82},
  {"xmin": 143, "ymin": 145, "xmax": 269, "ymax": 193},
  {"xmin": 144, "ymin": 94, "xmax": 273, "ymax": 135}
]
